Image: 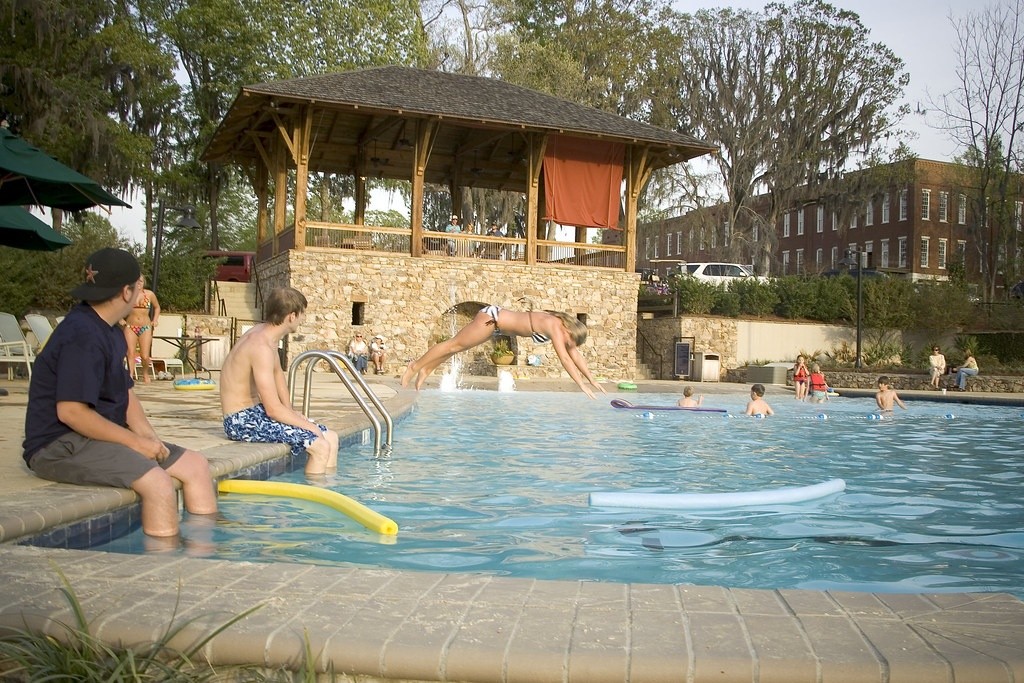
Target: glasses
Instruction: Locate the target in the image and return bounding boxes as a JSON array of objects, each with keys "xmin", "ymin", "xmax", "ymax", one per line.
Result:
[
  {"xmin": 356, "ymin": 336, "xmax": 362, "ymax": 338},
  {"xmin": 932, "ymin": 349, "xmax": 938, "ymax": 351}
]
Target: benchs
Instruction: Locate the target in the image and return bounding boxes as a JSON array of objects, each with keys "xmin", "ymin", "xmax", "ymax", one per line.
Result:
[{"xmin": 368, "ymin": 361, "xmax": 403, "ymax": 375}]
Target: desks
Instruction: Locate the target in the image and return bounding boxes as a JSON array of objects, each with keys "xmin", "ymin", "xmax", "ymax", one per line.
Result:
[{"xmin": 152, "ymin": 336, "xmax": 220, "ymax": 379}]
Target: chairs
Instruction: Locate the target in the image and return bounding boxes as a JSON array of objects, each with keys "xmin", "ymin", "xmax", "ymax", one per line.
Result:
[
  {"xmin": 0, "ymin": 312, "xmax": 184, "ymax": 380},
  {"xmin": 315, "ymin": 236, "xmax": 376, "ymax": 251}
]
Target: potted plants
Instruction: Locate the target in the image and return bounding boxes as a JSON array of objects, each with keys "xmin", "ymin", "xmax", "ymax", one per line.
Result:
[{"xmin": 491, "ymin": 339, "xmax": 515, "ymax": 365}]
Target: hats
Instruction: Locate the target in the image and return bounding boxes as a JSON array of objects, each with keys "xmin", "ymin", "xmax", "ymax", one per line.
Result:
[
  {"xmin": 73, "ymin": 247, "xmax": 141, "ymax": 301},
  {"xmin": 375, "ymin": 335, "xmax": 384, "ymax": 340}
]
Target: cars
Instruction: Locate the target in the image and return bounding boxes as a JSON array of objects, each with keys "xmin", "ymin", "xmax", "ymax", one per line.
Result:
[
  {"xmin": 674, "ymin": 264, "xmax": 778, "ymax": 290},
  {"xmin": 196, "ymin": 251, "xmax": 257, "ymax": 283}
]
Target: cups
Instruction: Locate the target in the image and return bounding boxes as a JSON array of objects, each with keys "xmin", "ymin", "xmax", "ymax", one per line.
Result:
[
  {"xmin": 941, "ymin": 388, "xmax": 947, "ymax": 395},
  {"xmin": 198, "ymin": 333, "xmax": 202, "ymax": 338}
]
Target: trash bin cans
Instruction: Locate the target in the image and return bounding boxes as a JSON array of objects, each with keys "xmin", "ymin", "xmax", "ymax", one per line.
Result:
[{"xmin": 694, "ymin": 352, "xmax": 720, "ymax": 383}]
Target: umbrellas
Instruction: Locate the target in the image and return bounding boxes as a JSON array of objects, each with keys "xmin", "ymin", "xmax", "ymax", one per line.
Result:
[{"xmin": 0, "ymin": 128, "xmax": 133, "ymax": 251}]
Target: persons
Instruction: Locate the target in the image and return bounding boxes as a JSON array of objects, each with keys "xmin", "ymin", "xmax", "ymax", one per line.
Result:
[
  {"xmin": 401, "ymin": 306, "xmax": 609, "ymax": 401},
  {"xmin": 929, "ymin": 346, "xmax": 946, "ymax": 389},
  {"xmin": 370, "ymin": 336, "xmax": 389, "ymax": 373},
  {"xmin": 875, "ymin": 376, "xmax": 907, "ymax": 411},
  {"xmin": 676, "ymin": 385, "xmax": 702, "ymax": 407},
  {"xmin": 348, "ymin": 333, "xmax": 369, "ymax": 374},
  {"xmin": 219, "ymin": 285, "xmax": 339, "ymax": 474},
  {"xmin": 793, "ymin": 355, "xmax": 830, "ymax": 404},
  {"xmin": 950, "ymin": 348, "xmax": 979, "ymax": 392},
  {"xmin": 119, "ymin": 273, "xmax": 161, "ymax": 385},
  {"xmin": 746, "ymin": 384, "xmax": 774, "ymax": 416},
  {"xmin": 22, "ymin": 248, "xmax": 217, "ymax": 536}
]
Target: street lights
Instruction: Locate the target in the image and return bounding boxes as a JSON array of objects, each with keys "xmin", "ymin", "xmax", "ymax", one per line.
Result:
[
  {"xmin": 148, "ymin": 197, "xmax": 202, "ymax": 362},
  {"xmin": 838, "ymin": 245, "xmax": 866, "ymax": 369}
]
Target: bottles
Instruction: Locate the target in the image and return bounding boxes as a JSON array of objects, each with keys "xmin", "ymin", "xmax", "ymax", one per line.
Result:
[{"xmin": 195, "ymin": 326, "xmax": 199, "ymax": 338}]
